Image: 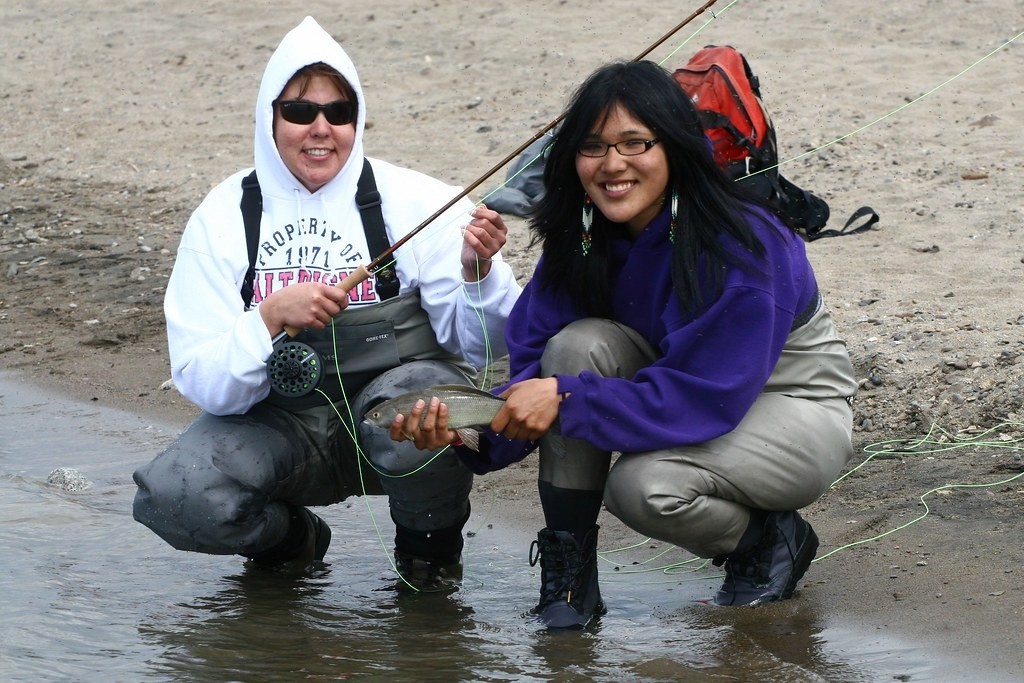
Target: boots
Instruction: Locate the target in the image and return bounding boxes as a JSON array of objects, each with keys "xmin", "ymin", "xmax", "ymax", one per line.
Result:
[
  {"xmin": 387, "ymin": 499, "xmax": 470, "ymax": 598},
  {"xmin": 712, "ymin": 506, "xmax": 818, "ymax": 608},
  {"xmin": 255, "ymin": 508, "xmax": 333, "ymax": 580},
  {"xmin": 529, "ymin": 523, "xmax": 606, "ymax": 638}
]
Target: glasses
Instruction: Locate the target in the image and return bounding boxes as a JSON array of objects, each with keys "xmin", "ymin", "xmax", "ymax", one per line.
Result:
[
  {"xmin": 576, "ymin": 135, "xmax": 661, "ymax": 157},
  {"xmin": 273, "ymin": 99, "xmax": 357, "ymax": 125}
]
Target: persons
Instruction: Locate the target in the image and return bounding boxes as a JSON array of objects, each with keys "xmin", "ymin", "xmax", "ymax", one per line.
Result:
[
  {"xmin": 391, "ymin": 60, "xmax": 859, "ymax": 637},
  {"xmin": 133, "ymin": 15, "xmax": 522, "ymax": 593}
]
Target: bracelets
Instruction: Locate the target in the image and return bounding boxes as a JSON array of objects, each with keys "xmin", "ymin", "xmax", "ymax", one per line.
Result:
[{"xmin": 561, "ymin": 391, "xmax": 567, "ymax": 402}]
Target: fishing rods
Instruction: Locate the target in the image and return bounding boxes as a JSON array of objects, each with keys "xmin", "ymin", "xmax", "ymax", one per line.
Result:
[{"xmin": 263, "ymin": 0, "xmax": 719, "ymax": 402}]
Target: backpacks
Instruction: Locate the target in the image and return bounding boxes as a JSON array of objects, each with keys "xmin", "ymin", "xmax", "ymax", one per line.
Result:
[{"xmin": 670, "ymin": 43, "xmax": 829, "ymax": 237}]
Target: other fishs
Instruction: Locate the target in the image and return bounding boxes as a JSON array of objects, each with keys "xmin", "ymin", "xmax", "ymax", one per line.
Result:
[{"xmin": 362, "ymin": 383, "xmax": 506, "ymax": 452}]
